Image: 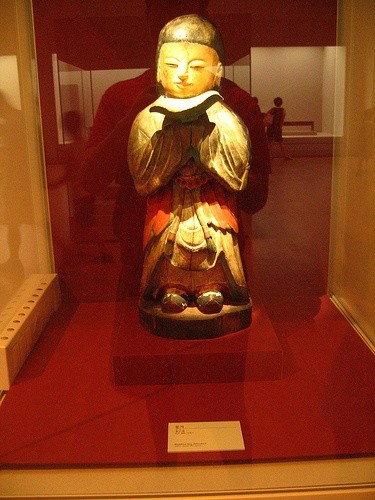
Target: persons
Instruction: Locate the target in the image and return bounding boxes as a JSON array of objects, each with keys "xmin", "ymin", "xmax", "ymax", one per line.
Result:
[
  {"xmin": 127, "ymin": 13, "xmax": 254, "ymax": 315},
  {"xmin": 76, "ymin": 0, "xmax": 271, "ymax": 465},
  {"xmin": 264, "ymin": 96, "xmax": 293, "ymax": 159},
  {"xmin": 252, "ymin": 96, "xmax": 267, "ymax": 120},
  {"xmin": 48, "ymin": 110, "xmax": 114, "ymax": 264}
]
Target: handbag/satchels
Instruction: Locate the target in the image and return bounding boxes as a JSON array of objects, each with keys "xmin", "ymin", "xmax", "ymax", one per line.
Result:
[{"xmin": 262, "ymin": 113, "xmax": 273, "ymax": 126}]
